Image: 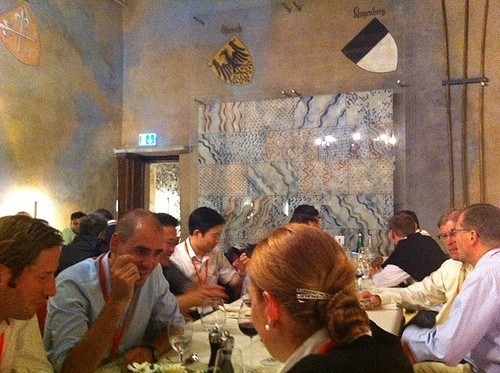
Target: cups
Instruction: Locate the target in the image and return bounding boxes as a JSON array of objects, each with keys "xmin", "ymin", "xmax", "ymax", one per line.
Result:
[
  {"xmin": 241, "ymin": 275, "xmax": 252, "ymax": 308},
  {"xmin": 213, "ymin": 348, "xmax": 245, "ymax": 373}
]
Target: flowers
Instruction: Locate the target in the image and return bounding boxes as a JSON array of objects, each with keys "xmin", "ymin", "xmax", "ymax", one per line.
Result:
[{"xmin": 127, "ymin": 361, "xmax": 221, "ymax": 373}]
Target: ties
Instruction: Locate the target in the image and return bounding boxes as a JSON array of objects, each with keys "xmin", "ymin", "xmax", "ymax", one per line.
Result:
[{"xmin": 457, "ymin": 268, "xmax": 467, "ymax": 294}]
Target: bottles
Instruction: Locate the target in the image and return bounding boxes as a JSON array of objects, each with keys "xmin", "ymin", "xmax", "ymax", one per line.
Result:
[
  {"xmin": 207, "ymin": 329, "xmax": 235, "ymax": 373},
  {"xmin": 356, "ymin": 232, "xmax": 364, "ymax": 254},
  {"xmin": 365, "ymin": 236, "xmax": 376, "ymax": 262}
]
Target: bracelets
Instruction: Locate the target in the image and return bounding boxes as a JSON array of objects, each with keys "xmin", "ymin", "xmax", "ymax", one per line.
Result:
[
  {"xmin": 237, "ymin": 272, "xmax": 245, "ymax": 279},
  {"xmin": 142, "ymin": 345, "xmax": 155, "ymax": 359}
]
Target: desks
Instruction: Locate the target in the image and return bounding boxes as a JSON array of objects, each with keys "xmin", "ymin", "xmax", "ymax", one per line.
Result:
[{"xmin": 154, "ymin": 279, "xmax": 403, "ymax": 373}]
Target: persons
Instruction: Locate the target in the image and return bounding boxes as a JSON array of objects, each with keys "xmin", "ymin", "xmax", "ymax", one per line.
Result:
[
  {"xmin": 15, "ymin": 208, "xmax": 118, "ymax": 278},
  {"xmin": 358, "ymin": 207, "xmax": 473, "ymax": 329},
  {"xmin": 0, "ymin": 215, "xmax": 65, "ymax": 373},
  {"xmin": 246, "ymin": 223, "xmax": 415, "ymax": 373},
  {"xmin": 401, "ymin": 203, "xmax": 500, "ymax": 373},
  {"xmin": 169, "ymin": 208, "xmax": 250, "ymax": 319},
  {"xmin": 370, "ymin": 210, "xmax": 450, "ymax": 329},
  {"xmin": 241, "ymin": 205, "xmax": 321, "ymax": 298},
  {"xmin": 142, "ymin": 213, "xmax": 229, "ymax": 347},
  {"xmin": 43, "ymin": 208, "xmax": 186, "ymax": 373}
]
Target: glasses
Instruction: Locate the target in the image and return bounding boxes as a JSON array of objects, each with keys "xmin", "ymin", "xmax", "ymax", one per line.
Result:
[
  {"xmin": 162, "ymin": 240, "xmax": 179, "ymax": 247},
  {"xmin": 437, "ymin": 231, "xmax": 456, "ymax": 239},
  {"xmin": 451, "ymin": 228, "xmax": 481, "ymax": 238}
]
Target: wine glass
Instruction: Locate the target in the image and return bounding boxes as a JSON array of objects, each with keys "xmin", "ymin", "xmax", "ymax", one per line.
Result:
[
  {"xmin": 168, "ymin": 317, "xmax": 194, "ymax": 368},
  {"xmin": 351, "ymin": 253, "xmax": 369, "ymax": 292},
  {"xmin": 237, "ymin": 299, "xmax": 259, "ymax": 346},
  {"xmin": 201, "ymin": 298, "xmax": 226, "ymax": 344}
]
